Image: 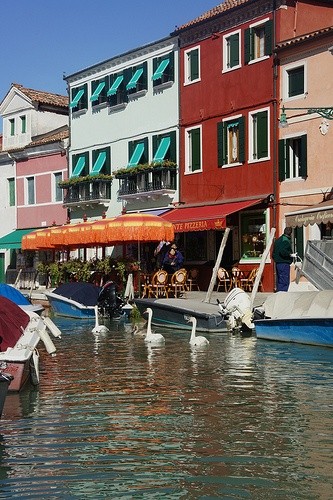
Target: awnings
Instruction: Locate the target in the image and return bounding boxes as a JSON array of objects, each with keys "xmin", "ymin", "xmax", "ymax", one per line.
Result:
[
  {"xmin": 0, "ymin": 227, "xmax": 44, "ymax": 249},
  {"xmin": 284, "ymin": 199, "xmax": 333, "ymax": 228},
  {"xmin": 158, "ymin": 198, "xmax": 263, "ymax": 233}
]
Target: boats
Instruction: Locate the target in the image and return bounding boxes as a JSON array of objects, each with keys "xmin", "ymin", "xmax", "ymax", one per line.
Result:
[
  {"xmin": 41, "ymin": 285, "xmax": 132, "ymax": 321},
  {"xmin": 241, "ymin": 287, "xmax": 333, "ymax": 349},
  {"xmin": 129, "ymin": 286, "xmax": 257, "ymax": 342},
  {"xmin": 0, "ymin": 296, "xmax": 47, "ymax": 393},
  {"xmin": 0, "ymin": 283, "xmax": 45, "ymax": 323}
]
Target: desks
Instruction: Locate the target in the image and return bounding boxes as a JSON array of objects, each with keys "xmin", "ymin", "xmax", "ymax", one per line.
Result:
[{"xmin": 222, "ymin": 270, "xmax": 251, "ymax": 290}]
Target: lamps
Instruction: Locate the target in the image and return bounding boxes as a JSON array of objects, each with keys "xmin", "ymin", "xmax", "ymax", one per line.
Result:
[{"xmin": 280, "ymin": 104, "xmax": 333, "ymax": 128}]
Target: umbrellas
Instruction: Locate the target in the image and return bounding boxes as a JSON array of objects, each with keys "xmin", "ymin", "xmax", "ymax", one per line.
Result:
[{"xmin": 21, "ymin": 211, "xmax": 175, "ymax": 264}]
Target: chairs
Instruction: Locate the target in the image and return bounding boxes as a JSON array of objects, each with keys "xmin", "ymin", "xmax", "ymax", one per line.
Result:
[
  {"xmin": 230, "ymin": 267, "xmax": 245, "ymax": 290},
  {"xmin": 215, "ymin": 267, "xmax": 231, "ymax": 293},
  {"xmin": 244, "ymin": 267, "xmax": 264, "ymax": 292},
  {"xmin": 146, "ymin": 268, "xmax": 199, "ymax": 300}
]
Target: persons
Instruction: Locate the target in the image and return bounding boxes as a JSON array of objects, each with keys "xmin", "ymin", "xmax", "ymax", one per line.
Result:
[
  {"xmin": 272, "ymin": 226, "xmax": 297, "ymax": 292},
  {"xmin": 163, "ymin": 248, "xmax": 178, "ymax": 274},
  {"xmin": 165, "ymin": 243, "xmax": 184, "ymax": 270}
]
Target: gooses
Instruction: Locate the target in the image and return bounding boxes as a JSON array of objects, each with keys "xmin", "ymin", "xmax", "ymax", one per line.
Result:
[
  {"xmin": 91, "ymin": 305, "xmax": 109, "ymax": 333},
  {"xmin": 187, "ymin": 317, "xmax": 210, "ymax": 346},
  {"xmin": 143, "ymin": 308, "xmax": 166, "ymax": 343}
]
